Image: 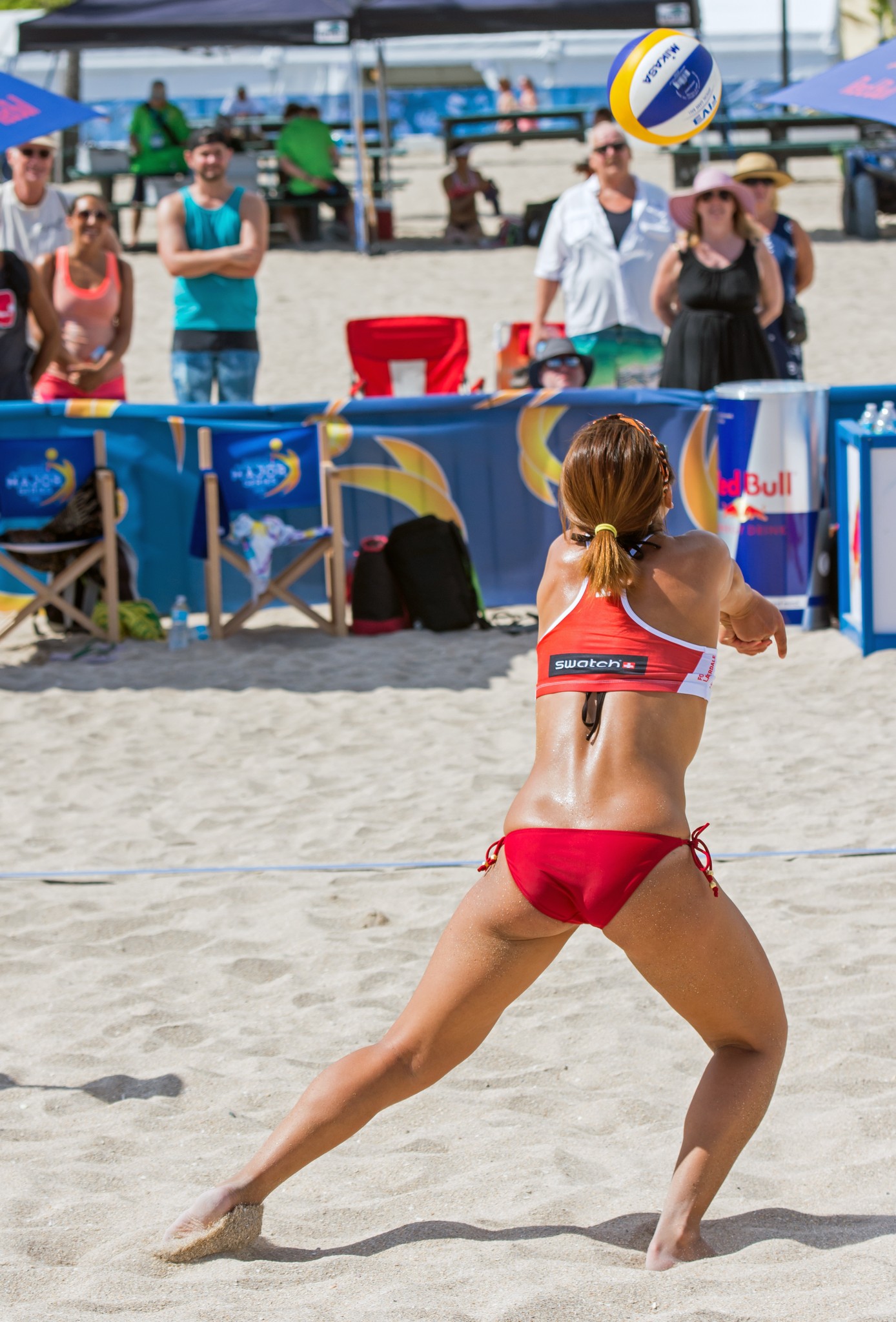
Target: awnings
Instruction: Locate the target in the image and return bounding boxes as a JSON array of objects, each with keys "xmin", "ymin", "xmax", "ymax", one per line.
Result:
[{"xmin": 18, "ymin": 0, "xmax": 703, "ymax": 60}]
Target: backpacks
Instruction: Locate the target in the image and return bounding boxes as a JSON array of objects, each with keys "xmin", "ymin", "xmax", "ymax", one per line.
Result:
[
  {"xmin": 386, "ymin": 515, "xmax": 494, "ymax": 632},
  {"xmin": 352, "ymin": 540, "xmax": 412, "ymax": 636},
  {"xmin": 44, "ymin": 530, "xmax": 140, "ymax": 633}
]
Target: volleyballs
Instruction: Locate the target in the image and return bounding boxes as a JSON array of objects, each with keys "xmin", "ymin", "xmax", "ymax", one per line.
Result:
[{"xmin": 607, "ymin": 28, "xmax": 722, "ymax": 146}]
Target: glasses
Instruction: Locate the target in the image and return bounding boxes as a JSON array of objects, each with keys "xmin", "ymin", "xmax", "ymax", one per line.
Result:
[
  {"xmin": 72, "ymin": 210, "xmax": 110, "ymax": 222},
  {"xmin": 702, "ymin": 191, "xmax": 729, "ymax": 201},
  {"xmin": 16, "ymin": 147, "xmax": 55, "ymax": 158},
  {"xmin": 545, "ymin": 357, "xmax": 582, "ymax": 368},
  {"xmin": 740, "ymin": 177, "xmax": 772, "ymax": 187},
  {"xmin": 593, "ymin": 141, "xmax": 626, "ymax": 153}
]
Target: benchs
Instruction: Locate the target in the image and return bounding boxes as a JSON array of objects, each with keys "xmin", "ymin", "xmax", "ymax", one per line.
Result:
[{"xmin": 106, "ymin": 193, "xmax": 290, "ymax": 210}]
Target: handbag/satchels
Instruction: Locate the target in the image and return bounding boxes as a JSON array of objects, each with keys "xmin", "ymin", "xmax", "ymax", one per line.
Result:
[
  {"xmin": 522, "ymin": 198, "xmax": 561, "ymax": 247},
  {"xmin": 91, "ymin": 598, "xmax": 166, "ymax": 642}
]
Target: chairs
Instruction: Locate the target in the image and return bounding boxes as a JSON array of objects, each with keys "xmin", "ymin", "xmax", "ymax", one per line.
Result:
[
  {"xmin": 344, "ymin": 311, "xmax": 483, "ymax": 398},
  {"xmin": 0, "ymin": 429, "xmax": 124, "ymax": 647},
  {"xmin": 471, "ymin": 319, "xmax": 569, "ymax": 393},
  {"xmin": 196, "ymin": 421, "xmax": 358, "ymax": 641}
]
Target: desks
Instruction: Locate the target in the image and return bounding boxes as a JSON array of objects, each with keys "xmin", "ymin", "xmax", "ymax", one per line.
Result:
[{"xmin": 243, "ymin": 138, "xmax": 303, "ymax": 242}]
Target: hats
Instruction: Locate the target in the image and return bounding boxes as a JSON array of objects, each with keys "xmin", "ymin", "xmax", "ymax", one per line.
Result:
[
  {"xmin": 187, "ymin": 127, "xmax": 231, "ymax": 150},
  {"xmin": 668, "ymin": 167, "xmax": 756, "ymax": 231},
  {"xmin": 529, "ymin": 338, "xmax": 594, "ymax": 389},
  {"xmin": 730, "ymin": 152, "xmax": 795, "ymax": 188}
]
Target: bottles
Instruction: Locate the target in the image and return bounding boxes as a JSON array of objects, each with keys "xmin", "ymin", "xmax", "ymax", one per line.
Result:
[
  {"xmin": 187, "ymin": 626, "xmax": 210, "ymax": 643},
  {"xmin": 169, "ymin": 595, "xmax": 188, "ymax": 653},
  {"xmin": 859, "ymin": 402, "xmax": 878, "ymax": 431},
  {"xmin": 873, "ymin": 400, "xmax": 896, "ymax": 435}
]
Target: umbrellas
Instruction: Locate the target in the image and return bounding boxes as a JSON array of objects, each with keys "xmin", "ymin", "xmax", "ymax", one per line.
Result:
[
  {"xmin": 760, "ymin": 37, "xmax": 895, "ymax": 131},
  {"xmin": 0, "ymin": 70, "xmax": 113, "ymax": 156}
]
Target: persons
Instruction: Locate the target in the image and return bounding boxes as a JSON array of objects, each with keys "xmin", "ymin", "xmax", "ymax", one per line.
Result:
[
  {"xmin": 513, "ymin": 337, "xmax": 595, "ymax": 392},
  {"xmin": 129, "ymin": 80, "xmax": 192, "ymax": 250},
  {"xmin": 0, "ymin": 251, "xmax": 62, "ymax": 401},
  {"xmin": 0, "ymin": 134, "xmax": 78, "ymax": 361},
  {"xmin": 28, "ymin": 194, "xmax": 135, "ymax": 403},
  {"xmin": 157, "ymin": 124, "xmax": 268, "ymax": 406},
  {"xmin": 440, "ymin": 142, "xmax": 496, "ymax": 238},
  {"xmin": 532, "ymin": 119, "xmax": 679, "ymax": 392},
  {"xmin": 222, "ymin": 83, "xmax": 263, "ymax": 139},
  {"xmin": 731, "ymin": 151, "xmax": 815, "ymax": 385},
  {"xmin": 498, "ymin": 75, "xmax": 540, "ymax": 136},
  {"xmin": 161, "ymin": 412, "xmax": 788, "ymax": 1272},
  {"xmin": 278, "ymin": 102, "xmax": 359, "ymax": 248},
  {"xmin": 651, "ymin": 164, "xmax": 785, "ymax": 392}
]
